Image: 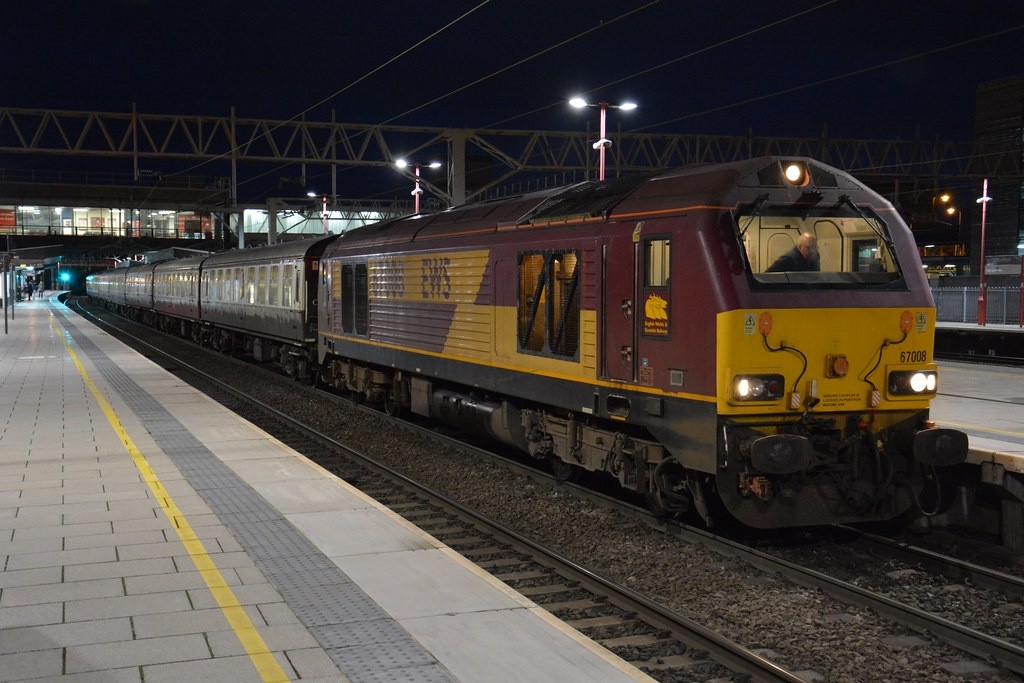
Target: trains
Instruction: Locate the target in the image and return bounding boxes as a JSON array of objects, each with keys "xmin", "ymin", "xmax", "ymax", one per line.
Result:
[{"xmin": 86, "ymin": 155, "xmax": 969, "ymax": 537}]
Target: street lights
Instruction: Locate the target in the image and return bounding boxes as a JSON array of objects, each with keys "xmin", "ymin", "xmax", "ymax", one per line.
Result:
[
  {"xmin": 572, "ymin": 99, "xmax": 637, "ymax": 180},
  {"xmin": 307, "ymin": 192, "xmax": 340, "ymax": 233},
  {"xmin": 397, "ymin": 160, "xmax": 441, "ymax": 214}
]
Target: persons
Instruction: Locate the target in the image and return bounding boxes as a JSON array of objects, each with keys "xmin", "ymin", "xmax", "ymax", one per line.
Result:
[
  {"xmin": 764, "ymin": 233, "xmax": 821, "ymax": 273},
  {"xmin": 38, "ymin": 281, "xmax": 44, "ymax": 298},
  {"xmin": 28, "ymin": 282, "xmax": 32, "ymax": 301}
]
